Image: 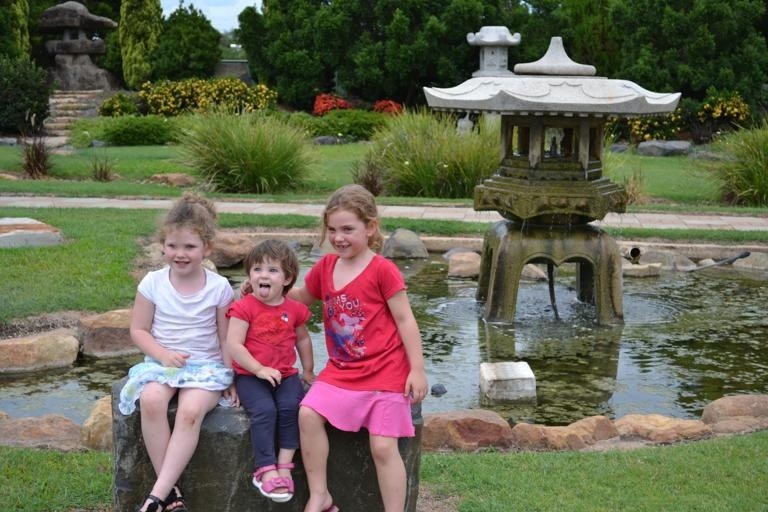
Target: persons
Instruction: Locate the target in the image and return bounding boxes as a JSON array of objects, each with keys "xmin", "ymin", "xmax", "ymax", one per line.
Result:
[
  {"xmin": 223, "ymin": 238, "xmax": 320, "ymax": 504},
  {"xmin": 239, "ymin": 182, "xmax": 430, "ymax": 510},
  {"xmin": 125, "ymin": 190, "xmax": 240, "ymax": 511}
]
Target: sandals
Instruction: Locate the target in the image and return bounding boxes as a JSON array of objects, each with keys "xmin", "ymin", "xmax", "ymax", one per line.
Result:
[
  {"xmin": 253, "ymin": 463, "xmax": 296, "ymax": 503},
  {"xmin": 141, "ymin": 482, "xmax": 187, "ymax": 512}
]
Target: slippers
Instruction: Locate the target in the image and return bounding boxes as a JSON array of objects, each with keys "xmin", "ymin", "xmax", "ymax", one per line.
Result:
[{"xmin": 305, "ymin": 498, "xmax": 339, "ymax": 512}]
